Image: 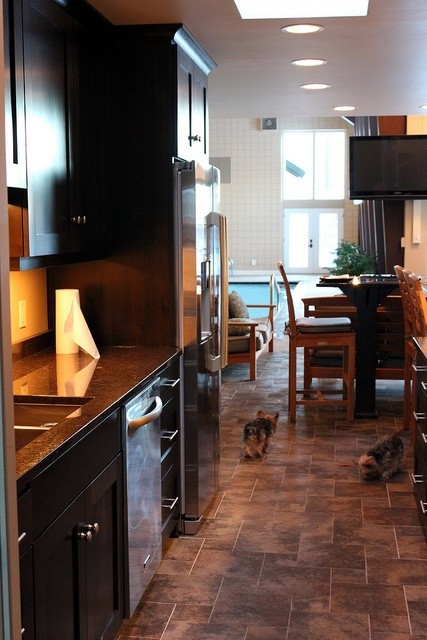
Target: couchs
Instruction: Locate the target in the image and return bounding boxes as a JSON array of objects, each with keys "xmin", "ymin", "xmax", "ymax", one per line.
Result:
[{"xmin": 227, "ymin": 291, "xmax": 275, "ymax": 382}]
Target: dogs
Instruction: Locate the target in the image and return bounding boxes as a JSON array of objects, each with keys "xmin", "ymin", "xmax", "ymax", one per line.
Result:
[
  {"xmin": 240, "ymin": 409, "xmax": 278, "ymax": 457},
  {"xmin": 351, "ymin": 427, "xmax": 410, "ymax": 481}
]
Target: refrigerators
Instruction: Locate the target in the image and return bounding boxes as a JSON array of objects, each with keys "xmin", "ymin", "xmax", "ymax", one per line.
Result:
[{"xmin": 46, "ymin": 158, "xmax": 227, "ymax": 538}]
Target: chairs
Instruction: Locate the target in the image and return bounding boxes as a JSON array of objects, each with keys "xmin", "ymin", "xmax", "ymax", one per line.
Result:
[
  {"xmin": 393, "ymin": 265, "xmax": 427, "ymax": 448},
  {"xmin": 276, "ymin": 258, "xmax": 356, "ymax": 424}
]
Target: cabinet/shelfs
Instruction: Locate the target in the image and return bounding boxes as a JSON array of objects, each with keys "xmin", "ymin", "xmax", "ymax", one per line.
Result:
[
  {"xmin": 411, "ymin": 336, "xmax": 427, "ymax": 543},
  {"xmin": 0, "ymin": 0, "xmax": 26, "ymax": 191},
  {"xmin": 111, "ymin": 22, "xmax": 217, "ymax": 164},
  {"xmin": 15, "ymin": 405, "xmax": 126, "ymax": 639},
  {"xmin": 158, "ymin": 348, "xmax": 180, "ymax": 557},
  {"xmin": 8, "ymin": 0, "xmax": 111, "ymax": 270}
]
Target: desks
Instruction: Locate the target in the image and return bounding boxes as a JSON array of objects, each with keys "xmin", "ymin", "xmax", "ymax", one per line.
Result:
[{"xmin": 316, "ymin": 274, "xmax": 422, "ymax": 419}]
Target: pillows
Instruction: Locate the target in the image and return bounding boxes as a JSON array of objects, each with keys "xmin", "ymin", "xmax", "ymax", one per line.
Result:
[
  {"xmin": 229, "ymin": 289, "xmax": 249, "ymax": 318},
  {"xmin": 228, "ymin": 317, "xmax": 259, "ymax": 336}
]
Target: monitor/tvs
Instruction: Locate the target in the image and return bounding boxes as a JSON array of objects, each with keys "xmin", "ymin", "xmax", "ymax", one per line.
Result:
[{"xmin": 350, "ymin": 134, "xmax": 427, "ymax": 200}]
